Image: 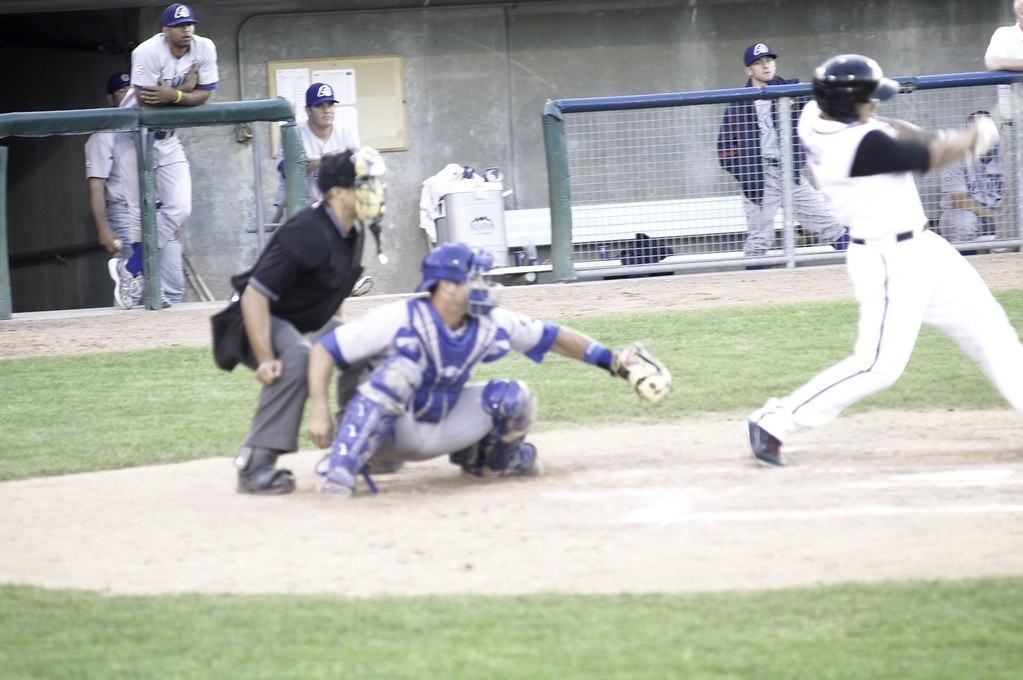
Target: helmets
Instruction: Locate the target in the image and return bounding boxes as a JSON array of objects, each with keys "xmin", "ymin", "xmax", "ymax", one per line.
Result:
[
  {"xmin": 415, "ymin": 243, "xmax": 473, "ymax": 293},
  {"xmin": 311, "ymin": 147, "xmax": 373, "ymax": 209},
  {"xmin": 812, "ymin": 54, "xmax": 900, "ymax": 118}
]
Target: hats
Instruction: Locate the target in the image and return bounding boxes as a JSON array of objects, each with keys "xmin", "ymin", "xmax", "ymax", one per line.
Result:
[
  {"xmin": 107, "ymin": 72, "xmax": 130, "ymax": 95},
  {"xmin": 307, "ymin": 82, "xmax": 339, "ymax": 106},
  {"xmin": 744, "ymin": 42, "xmax": 776, "ymax": 66},
  {"xmin": 162, "ymin": 4, "xmax": 199, "ymax": 27}
]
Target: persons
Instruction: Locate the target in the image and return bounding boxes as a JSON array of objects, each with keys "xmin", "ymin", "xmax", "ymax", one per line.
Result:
[
  {"xmin": 84, "ymin": 72, "xmax": 183, "ymax": 309},
  {"xmin": 273, "ymin": 83, "xmax": 374, "ymax": 296},
  {"xmin": 302, "ymin": 242, "xmax": 672, "ymax": 497},
  {"xmin": 208, "ymin": 150, "xmax": 385, "ymax": 496},
  {"xmin": 717, "ymin": 45, "xmax": 853, "ymax": 268},
  {"xmin": 985, "ymin": 0, "xmax": 1023, "ymax": 242},
  {"xmin": 943, "ymin": 110, "xmax": 1004, "ymax": 245},
  {"xmin": 107, "ymin": 5, "xmax": 220, "ymax": 312},
  {"xmin": 748, "ymin": 54, "xmax": 1023, "ymax": 465}
]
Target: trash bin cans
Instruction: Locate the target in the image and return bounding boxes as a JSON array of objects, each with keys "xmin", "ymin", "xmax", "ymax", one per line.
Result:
[{"xmin": 430, "ymin": 189, "xmax": 509, "ymax": 269}]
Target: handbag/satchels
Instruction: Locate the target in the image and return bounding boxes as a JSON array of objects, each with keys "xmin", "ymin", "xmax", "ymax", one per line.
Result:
[{"xmin": 212, "ymin": 298, "xmax": 247, "ymax": 370}]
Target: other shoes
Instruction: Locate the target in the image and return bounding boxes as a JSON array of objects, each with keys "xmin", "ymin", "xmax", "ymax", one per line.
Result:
[
  {"xmin": 237, "ymin": 463, "xmax": 295, "ymax": 495},
  {"xmin": 316, "ymin": 465, "xmax": 356, "ymax": 498},
  {"xmin": 461, "ymin": 440, "xmax": 538, "ymax": 478}
]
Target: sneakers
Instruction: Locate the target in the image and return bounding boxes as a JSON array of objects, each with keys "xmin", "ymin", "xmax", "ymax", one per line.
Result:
[
  {"xmin": 108, "ymin": 256, "xmax": 142, "ymax": 309},
  {"xmin": 747, "ymin": 414, "xmax": 784, "ymax": 469},
  {"xmin": 346, "ymin": 276, "xmax": 373, "ymax": 296},
  {"xmin": 159, "ymin": 287, "xmax": 172, "ymax": 307}
]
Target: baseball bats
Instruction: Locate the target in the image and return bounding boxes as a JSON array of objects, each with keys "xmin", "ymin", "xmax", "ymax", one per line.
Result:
[
  {"xmin": 183, "ymin": 264, "xmax": 205, "ymax": 302},
  {"xmin": 183, "ymin": 251, "xmax": 217, "ymax": 301}
]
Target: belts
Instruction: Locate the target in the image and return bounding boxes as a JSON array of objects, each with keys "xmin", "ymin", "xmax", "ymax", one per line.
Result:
[
  {"xmin": 849, "ymin": 222, "xmax": 929, "ymax": 246},
  {"xmin": 137, "ymin": 126, "xmax": 175, "ymax": 139},
  {"xmin": 761, "ymin": 157, "xmax": 777, "ymax": 167}
]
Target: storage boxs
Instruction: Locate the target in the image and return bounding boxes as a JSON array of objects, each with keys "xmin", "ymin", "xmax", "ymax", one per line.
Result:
[{"xmin": 433, "ymin": 181, "xmax": 508, "ymax": 269}]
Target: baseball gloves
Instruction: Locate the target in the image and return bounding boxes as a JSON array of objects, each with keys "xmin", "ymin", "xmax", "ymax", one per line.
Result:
[{"xmin": 614, "ymin": 340, "xmax": 673, "ymax": 403}]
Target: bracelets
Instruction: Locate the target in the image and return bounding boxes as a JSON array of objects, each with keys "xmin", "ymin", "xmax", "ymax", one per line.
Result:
[{"xmin": 174, "ymin": 91, "xmax": 182, "ymax": 103}]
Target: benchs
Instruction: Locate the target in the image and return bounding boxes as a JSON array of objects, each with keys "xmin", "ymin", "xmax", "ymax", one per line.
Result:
[{"xmin": 428, "ymin": 193, "xmax": 834, "ymax": 282}]
